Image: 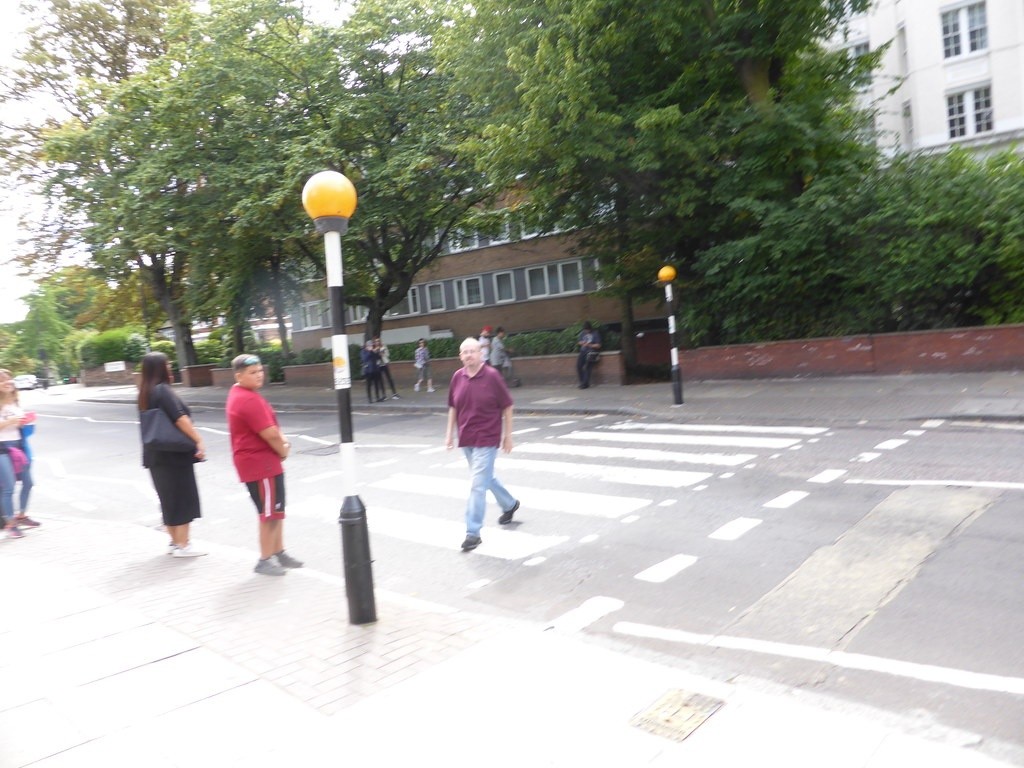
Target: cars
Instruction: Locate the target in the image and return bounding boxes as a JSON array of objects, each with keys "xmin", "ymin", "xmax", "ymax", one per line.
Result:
[{"xmin": 14, "ymin": 375, "xmax": 39, "ymax": 389}]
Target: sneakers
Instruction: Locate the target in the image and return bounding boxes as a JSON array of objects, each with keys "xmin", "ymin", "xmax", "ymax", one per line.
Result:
[
  {"xmin": 14, "ymin": 514, "xmax": 41, "ymax": 529},
  {"xmin": 276, "ymin": 551, "xmax": 302, "ymax": 568},
  {"xmin": 254, "ymin": 557, "xmax": 286, "ymax": 576},
  {"xmin": 168, "ymin": 540, "xmax": 208, "ymax": 558},
  {"xmin": 0, "ymin": 519, "xmax": 27, "ymax": 540},
  {"xmin": 461, "ymin": 535, "xmax": 482, "ymax": 549},
  {"xmin": 498, "ymin": 500, "xmax": 520, "ymax": 525}
]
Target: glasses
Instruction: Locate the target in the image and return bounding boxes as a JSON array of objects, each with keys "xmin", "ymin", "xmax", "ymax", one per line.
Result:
[{"xmin": 237, "ymin": 356, "xmax": 259, "ymax": 370}]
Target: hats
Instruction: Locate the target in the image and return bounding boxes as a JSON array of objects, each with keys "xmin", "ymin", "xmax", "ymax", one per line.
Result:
[
  {"xmin": 582, "ymin": 320, "xmax": 590, "ymax": 330},
  {"xmin": 483, "ymin": 326, "xmax": 492, "ymax": 331}
]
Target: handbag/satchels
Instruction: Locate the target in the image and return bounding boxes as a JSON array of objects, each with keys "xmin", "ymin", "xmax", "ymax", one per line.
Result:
[
  {"xmin": 586, "ymin": 350, "xmax": 600, "ymax": 362},
  {"xmin": 413, "ymin": 362, "xmax": 422, "ymax": 368},
  {"xmin": 140, "ymin": 387, "xmax": 196, "ymax": 455}
]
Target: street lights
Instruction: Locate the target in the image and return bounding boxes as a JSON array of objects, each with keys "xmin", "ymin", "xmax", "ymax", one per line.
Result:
[
  {"xmin": 658, "ymin": 264, "xmax": 684, "ymax": 405},
  {"xmin": 302, "ymin": 170, "xmax": 380, "ymax": 624}
]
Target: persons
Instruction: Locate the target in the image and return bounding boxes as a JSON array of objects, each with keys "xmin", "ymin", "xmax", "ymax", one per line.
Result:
[
  {"xmin": 139, "ymin": 351, "xmax": 209, "ymax": 558},
  {"xmin": 414, "ymin": 338, "xmax": 436, "ymax": 393},
  {"xmin": 445, "ymin": 337, "xmax": 520, "ymax": 548},
  {"xmin": 575, "ymin": 322, "xmax": 601, "ymax": 389},
  {"xmin": 479, "ymin": 325, "xmax": 514, "ymax": 387},
  {"xmin": 0, "ymin": 369, "xmax": 40, "ymax": 539},
  {"xmin": 225, "ymin": 353, "xmax": 305, "ymax": 575},
  {"xmin": 360, "ymin": 337, "xmax": 399, "ymax": 403}
]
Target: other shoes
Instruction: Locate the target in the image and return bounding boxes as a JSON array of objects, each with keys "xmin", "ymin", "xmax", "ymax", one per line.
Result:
[
  {"xmin": 426, "ymin": 388, "xmax": 435, "ymax": 392},
  {"xmin": 414, "ymin": 383, "xmax": 419, "ymax": 391}
]
